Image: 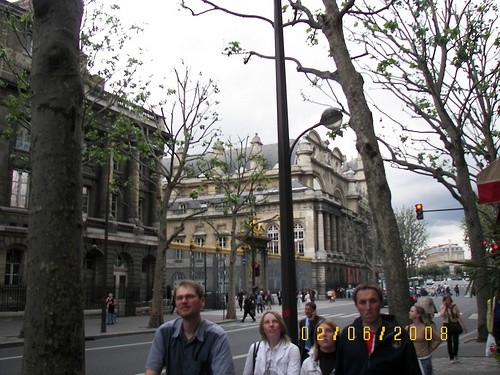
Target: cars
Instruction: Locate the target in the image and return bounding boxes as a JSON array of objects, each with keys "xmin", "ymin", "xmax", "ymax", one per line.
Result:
[
  {"xmin": 381, "ymin": 285, "xmax": 447, "ymax": 306},
  {"xmin": 426, "ymin": 278, "xmax": 434, "ymax": 286}
]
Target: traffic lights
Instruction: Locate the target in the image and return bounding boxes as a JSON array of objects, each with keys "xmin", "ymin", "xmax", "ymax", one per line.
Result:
[
  {"xmin": 415, "ymin": 204, "xmax": 424, "ymax": 220},
  {"xmin": 254, "ymin": 264, "xmax": 261, "ymax": 277}
]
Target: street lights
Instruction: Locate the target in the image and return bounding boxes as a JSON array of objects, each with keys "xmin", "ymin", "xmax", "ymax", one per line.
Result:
[{"xmin": 277, "ymin": 108, "xmax": 344, "ymax": 348}]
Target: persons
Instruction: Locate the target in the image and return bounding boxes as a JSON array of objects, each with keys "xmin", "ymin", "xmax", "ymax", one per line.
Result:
[
  {"xmin": 472, "ymin": 287, "xmax": 476, "ymax": 297},
  {"xmin": 440, "ymin": 294, "xmax": 469, "ymax": 364},
  {"xmin": 381, "ymin": 288, "xmax": 388, "ymax": 298},
  {"xmin": 105, "ymin": 293, "xmax": 118, "ymax": 325},
  {"xmin": 300, "ymin": 320, "xmax": 340, "ymax": 375},
  {"xmin": 169, "ymin": 295, "xmax": 176, "ymax": 315},
  {"xmin": 243, "ymin": 311, "xmax": 301, "ymax": 375},
  {"xmin": 408, "ymin": 282, "xmax": 460, "ymax": 297},
  {"xmin": 277, "ymin": 288, "xmax": 282, "ymax": 305},
  {"xmin": 335, "ymin": 281, "xmax": 423, "ymax": 375},
  {"xmin": 296, "ymin": 287, "xmax": 318, "ymax": 307},
  {"xmin": 405, "ymin": 306, "xmax": 444, "ymax": 375},
  {"xmin": 414, "ymin": 288, "xmax": 438, "ymax": 320},
  {"xmin": 327, "ymin": 283, "xmax": 356, "ymax": 303},
  {"xmin": 299, "ymin": 302, "xmax": 325, "ymax": 366},
  {"xmin": 130, "ymin": 291, "xmax": 137, "ymax": 316},
  {"xmin": 487, "ymin": 287, "xmax": 500, "ymax": 353},
  {"xmin": 145, "ymin": 279, "xmax": 236, "ymax": 375},
  {"xmin": 223, "ymin": 288, "xmax": 274, "ymax": 323}
]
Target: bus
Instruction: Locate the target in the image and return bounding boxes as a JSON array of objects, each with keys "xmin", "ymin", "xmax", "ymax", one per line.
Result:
[{"xmin": 408, "ymin": 276, "xmax": 424, "ymax": 289}]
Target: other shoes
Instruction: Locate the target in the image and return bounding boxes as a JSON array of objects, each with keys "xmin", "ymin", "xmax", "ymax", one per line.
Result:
[{"xmin": 450, "ymin": 356, "xmax": 459, "ymax": 363}]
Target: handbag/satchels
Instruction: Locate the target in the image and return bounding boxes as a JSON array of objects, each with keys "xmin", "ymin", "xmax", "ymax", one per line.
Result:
[
  {"xmin": 486, "ymin": 333, "xmax": 497, "ymax": 356},
  {"xmin": 458, "ymin": 321, "xmax": 463, "ymax": 335}
]
